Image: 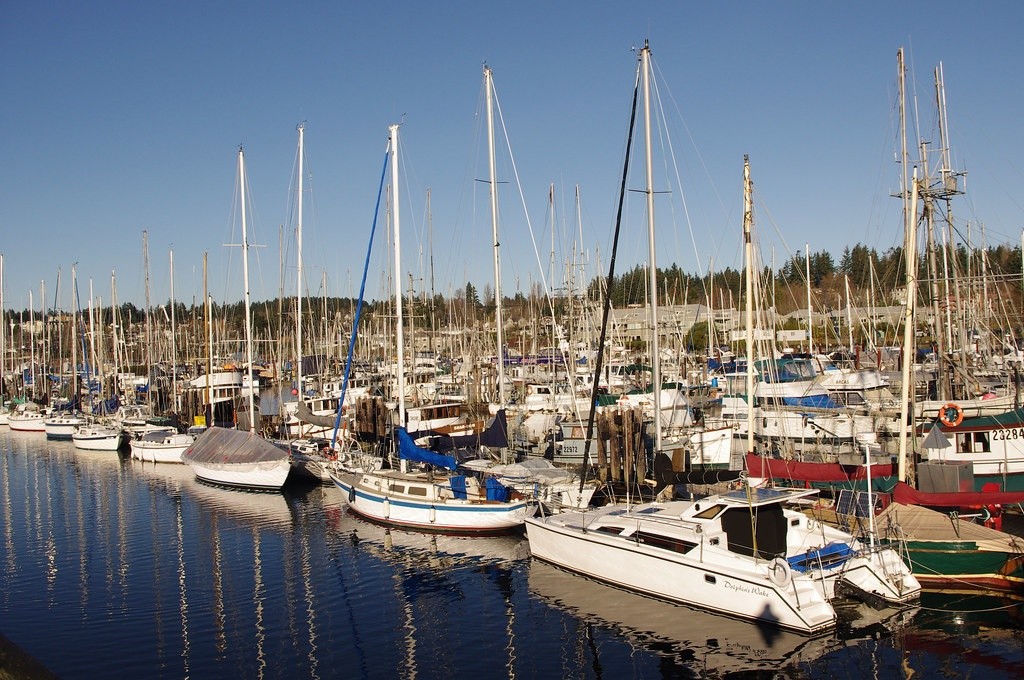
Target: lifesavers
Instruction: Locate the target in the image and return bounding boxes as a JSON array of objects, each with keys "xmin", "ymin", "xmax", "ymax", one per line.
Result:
[
  {"xmin": 940, "ymin": 403, "xmax": 963, "ymax": 427},
  {"xmin": 322, "ymin": 447, "xmax": 336, "ymax": 459}
]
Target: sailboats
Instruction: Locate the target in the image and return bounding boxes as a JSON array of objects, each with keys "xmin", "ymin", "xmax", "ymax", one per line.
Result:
[{"xmin": 6, "ymin": 35, "xmax": 1022, "ymax": 634}]
